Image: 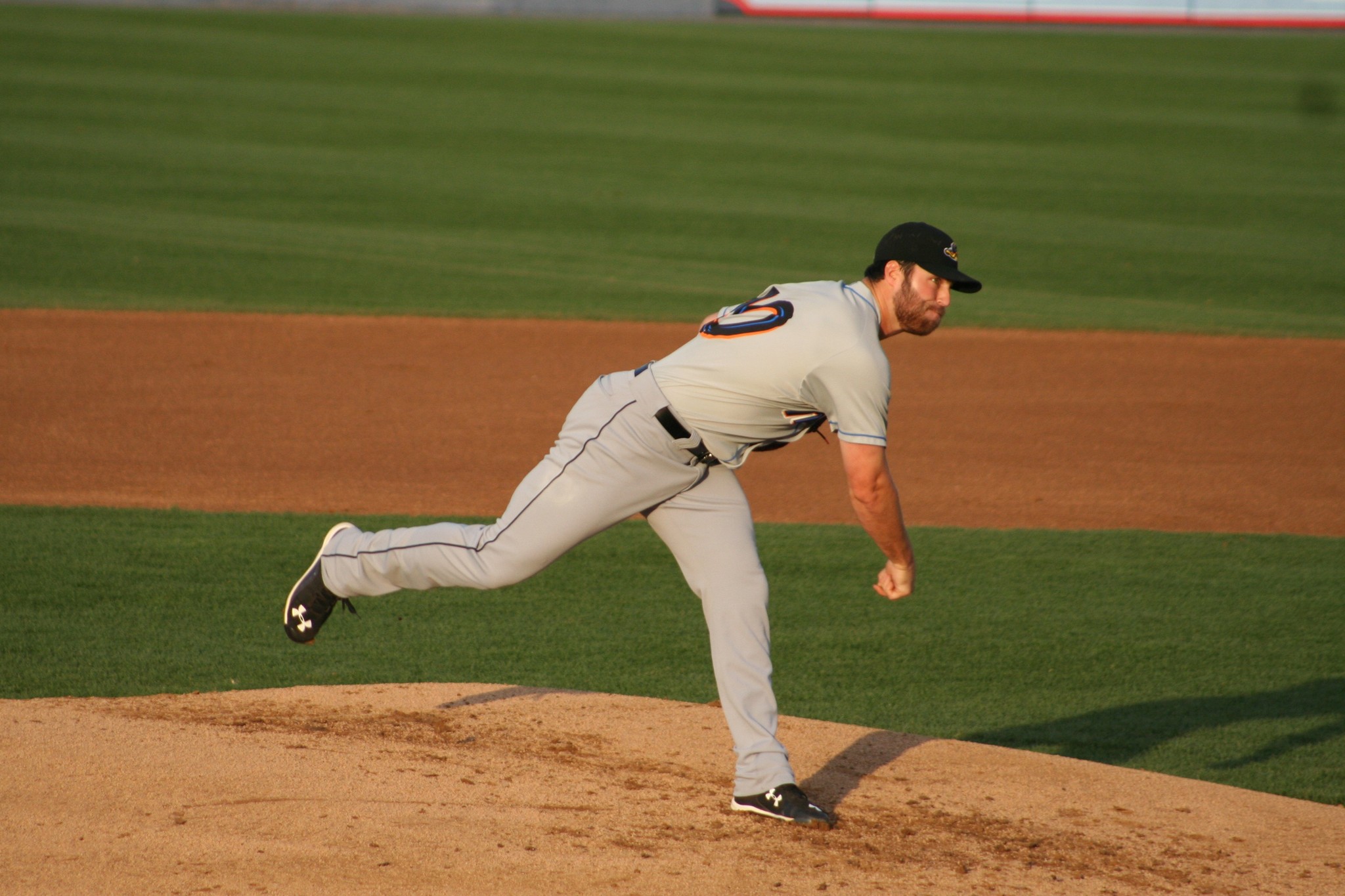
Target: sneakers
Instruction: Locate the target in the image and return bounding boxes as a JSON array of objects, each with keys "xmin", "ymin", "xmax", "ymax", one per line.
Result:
[
  {"xmin": 285, "ymin": 522, "xmax": 357, "ymax": 644},
  {"xmin": 731, "ymin": 782, "xmax": 832, "ymax": 828}
]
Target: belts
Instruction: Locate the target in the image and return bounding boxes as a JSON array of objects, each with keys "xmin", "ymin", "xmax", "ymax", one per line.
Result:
[{"xmin": 635, "ymin": 363, "xmax": 721, "ymax": 466}]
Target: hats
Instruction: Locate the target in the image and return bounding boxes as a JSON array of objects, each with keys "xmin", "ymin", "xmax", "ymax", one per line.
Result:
[{"xmin": 874, "ymin": 222, "xmax": 982, "ymax": 293}]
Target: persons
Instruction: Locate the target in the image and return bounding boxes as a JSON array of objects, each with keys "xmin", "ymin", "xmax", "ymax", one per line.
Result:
[{"xmin": 283, "ymin": 220, "xmax": 982, "ymax": 828}]
